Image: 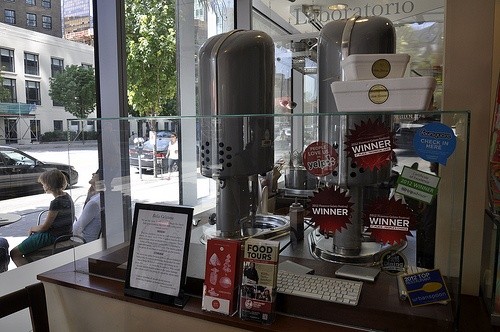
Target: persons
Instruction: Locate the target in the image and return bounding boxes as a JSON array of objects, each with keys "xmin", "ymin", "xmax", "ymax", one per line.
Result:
[
  {"xmin": 165, "ymin": 133, "xmax": 179, "ymax": 180},
  {"xmin": 70, "ymin": 169, "xmax": 101, "ymax": 244},
  {"xmin": 280, "ymin": 130, "xmax": 286, "ymax": 150},
  {"xmin": 10, "ymin": 169, "xmax": 75, "ymax": 267},
  {"xmin": 0, "ymin": 238, "xmax": 9, "ymax": 273},
  {"xmin": 259, "ymin": 289, "xmax": 271, "ymax": 301},
  {"xmin": 244, "ymin": 262, "xmax": 259, "ymax": 284}
]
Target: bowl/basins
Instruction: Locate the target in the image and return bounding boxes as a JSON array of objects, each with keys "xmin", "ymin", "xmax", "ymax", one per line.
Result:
[
  {"xmin": 331, "ymin": 76, "xmax": 436, "ymax": 111},
  {"xmin": 343, "ymin": 54, "xmax": 410, "ymax": 79}
]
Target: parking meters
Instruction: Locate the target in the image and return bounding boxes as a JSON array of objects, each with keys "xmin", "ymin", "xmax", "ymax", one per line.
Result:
[{"xmin": 134, "ymin": 137, "xmax": 145, "ymax": 181}]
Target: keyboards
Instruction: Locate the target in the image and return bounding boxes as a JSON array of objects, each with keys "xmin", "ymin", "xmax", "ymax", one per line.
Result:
[{"xmin": 275, "ymin": 269, "xmax": 363, "ymax": 306}]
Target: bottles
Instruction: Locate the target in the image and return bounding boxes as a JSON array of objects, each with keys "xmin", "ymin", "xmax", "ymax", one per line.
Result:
[{"xmin": 289, "ymin": 198, "xmax": 304, "ymax": 242}]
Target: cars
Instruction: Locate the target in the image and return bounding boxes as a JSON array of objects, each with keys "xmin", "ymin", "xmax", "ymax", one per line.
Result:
[
  {"xmin": 1, "ymin": 146, "xmax": 79, "ymax": 200},
  {"xmin": 129, "ymin": 137, "xmax": 178, "ymax": 174},
  {"xmin": 393, "ymin": 115, "xmax": 440, "ymax": 150}
]
